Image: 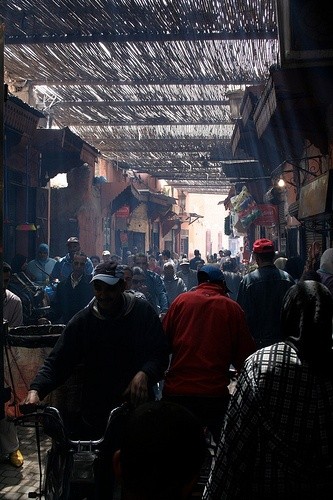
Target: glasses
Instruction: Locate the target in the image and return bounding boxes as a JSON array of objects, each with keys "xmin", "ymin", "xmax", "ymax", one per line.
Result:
[
  {"xmin": 132, "ymin": 279, "xmax": 147, "ymax": 284},
  {"xmin": 135, "ymin": 262, "xmax": 147, "ymax": 265}
]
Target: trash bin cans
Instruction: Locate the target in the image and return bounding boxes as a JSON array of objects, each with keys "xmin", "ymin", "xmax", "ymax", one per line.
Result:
[{"xmin": 4, "ymin": 325, "xmax": 66, "ymax": 418}]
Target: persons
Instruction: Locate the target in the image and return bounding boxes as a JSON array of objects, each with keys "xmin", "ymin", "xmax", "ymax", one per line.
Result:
[
  {"xmin": 114, "ymin": 401, "xmax": 204, "ymax": 500},
  {"xmin": 202, "ymin": 280, "xmax": 333, "ymax": 500},
  {"xmin": 20, "ymin": 262, "xmax": 168, "ymax": 500},
  {"xmin": 2, "ymin": 237, "xmax": 333, "ymax": 467}
]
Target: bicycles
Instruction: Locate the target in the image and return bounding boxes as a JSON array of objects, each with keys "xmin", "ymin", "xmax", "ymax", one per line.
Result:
[{"xmin": 29, "ymin": 400, "xmax": 131, "ymax": 500}]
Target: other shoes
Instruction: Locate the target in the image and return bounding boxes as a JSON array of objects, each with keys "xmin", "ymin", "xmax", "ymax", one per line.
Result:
[{"xmin": 10, "ymin": 450, "xmax": 24, "ymax": 467}]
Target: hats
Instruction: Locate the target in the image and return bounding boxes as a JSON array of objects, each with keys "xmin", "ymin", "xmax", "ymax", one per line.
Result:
[
  {"xmin": 90, "ymin": 261, "xmax": 122, "ymax": 286},
  {"xmin": 37, "ymin": 243, "xmax": 49, "ymax": 253},
  {"xmin": 197, "ymin": 266, "xmax": 226, "ymax": 285},
  {"xmin": 102, "ymin": 250, "xmax": 111, "ymax": 256},
  {"xmin": 252, "ymin": 238, "xmax": 275, "ymax": 254},
  {"xmin": 65, "ymin": 237, "xmax": 80, "ymax": 247},
  {"xmin": 179, "ymin": 258, "xmax": 190, "ymax": 266},
  {"xmin": 223, "ymin": 250, "xmax": 231, "ymax": 255}
]
